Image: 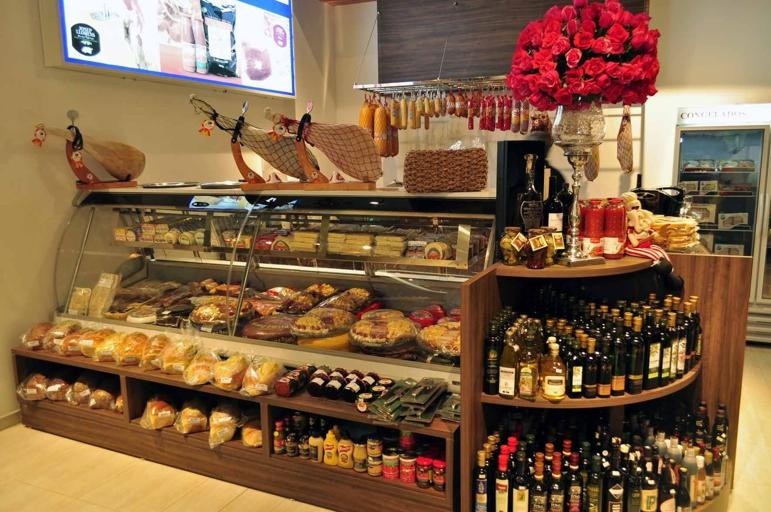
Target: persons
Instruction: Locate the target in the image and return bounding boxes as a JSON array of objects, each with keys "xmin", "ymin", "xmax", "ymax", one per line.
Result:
[{"xmin": 112, "ymin": 0, "xmax": 148, "ymax": 70}]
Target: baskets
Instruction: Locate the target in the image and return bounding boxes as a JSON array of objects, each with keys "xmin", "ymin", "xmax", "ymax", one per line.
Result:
[{"xmin": 403, "ymin": 147, "xmax": 490, "ymax": 193}]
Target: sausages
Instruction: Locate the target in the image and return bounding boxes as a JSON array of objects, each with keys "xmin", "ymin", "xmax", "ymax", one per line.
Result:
[{"xmin": 360, "ymin": 93, "xmax": 529, "ymax": 158}]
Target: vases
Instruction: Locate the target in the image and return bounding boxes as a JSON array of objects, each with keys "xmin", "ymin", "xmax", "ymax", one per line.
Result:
[{"xmin": 552, "ymin": 96, "xmax": 607, "ymax": 267}]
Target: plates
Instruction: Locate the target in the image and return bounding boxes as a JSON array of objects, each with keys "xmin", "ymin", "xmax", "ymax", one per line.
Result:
[
  {"xmin": 143, "ymin": 181, "xmax": 201, "ymax": 187},
  {"xmin": 202, "ymin": 182, "xmax": 242, "ymax": 188}
]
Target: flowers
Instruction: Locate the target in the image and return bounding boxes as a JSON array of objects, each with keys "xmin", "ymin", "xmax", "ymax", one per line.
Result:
[{"xmin": 505, "ymin": 0, "xmax": 662, "ymax": 111}]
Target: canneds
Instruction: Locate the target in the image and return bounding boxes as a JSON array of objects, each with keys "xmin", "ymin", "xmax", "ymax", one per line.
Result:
[
  {"xmin": 286, "ymin": 412, "xmax": 446, "ymax": 492},
  {"xmin": 500, "ymin": 226, "xmax": 558, "ymax": 268}
]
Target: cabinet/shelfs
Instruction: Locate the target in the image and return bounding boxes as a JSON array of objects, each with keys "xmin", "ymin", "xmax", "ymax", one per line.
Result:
[{"xmin": 460, "ymin": 252, "xmax": 753, "ymax": 511}]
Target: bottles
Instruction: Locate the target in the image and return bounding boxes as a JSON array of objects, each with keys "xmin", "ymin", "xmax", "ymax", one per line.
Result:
[
  {"xmin": 558, "ymin": 182, "xmax": 573, "ymax": 201},
  {"xmin": 274, "ymin": 413, "xmax": 354, "ymax": 468},
  {"xmin": 543, "ymin": 176, "xmax": 565, "ymax": 241},
  {"xmin": 475, "ymin": 282, "xmax": 728, "ymax": 512},
  {"xmin": 517, "ymin": 171, "xmax": 544, "ymax": 236},
  {"xmin": 579, "ymin": 198, "xmax": 627, "ymax": 260}
]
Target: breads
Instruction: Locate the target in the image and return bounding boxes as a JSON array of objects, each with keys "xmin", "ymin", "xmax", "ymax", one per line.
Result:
[
  {"xmin": 113, "ymin": 216, "xmax": 453, "ymax": 260},
  {"xmin": 16, "ymin": 361, "xmax": 123, "ymax": 413},
  {"xmin": 16, "ymin": 278, "xmax": 461, "ymax": 357},
  {"xmin": 23, "ymin": 321, "xmax": 288, "ymax": 398},
  {"xmin": 140, "ymin": 397, "xmax": 261, "ymax": 449}
]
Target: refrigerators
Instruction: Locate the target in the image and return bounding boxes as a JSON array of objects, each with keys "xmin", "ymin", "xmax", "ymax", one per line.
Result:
[{"xmin": 669, "ymin": 103, "xmax": 771, "ymax": 347}]
